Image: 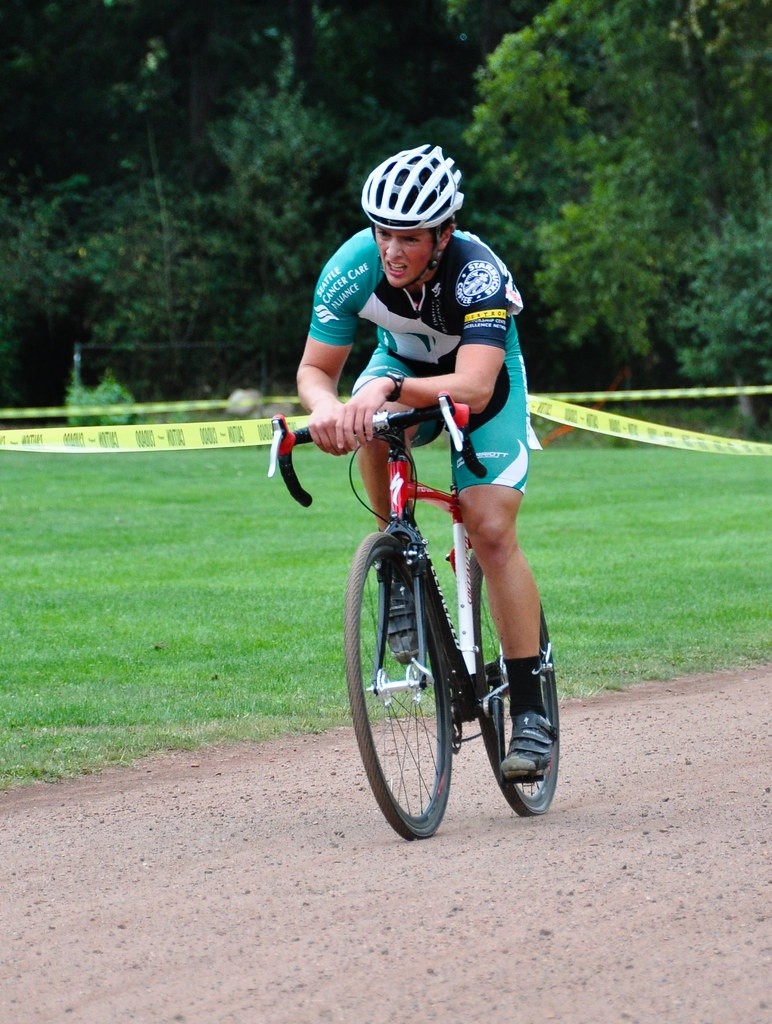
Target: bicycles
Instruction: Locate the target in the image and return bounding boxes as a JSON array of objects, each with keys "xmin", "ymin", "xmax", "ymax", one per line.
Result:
[{"xmin": 267, "ymin": 391, "xmax": 561, "ymax": 842}]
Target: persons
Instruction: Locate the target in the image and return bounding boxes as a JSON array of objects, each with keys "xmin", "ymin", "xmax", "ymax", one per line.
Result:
[{"xmin": 295, "ymin": 143, "xmax": 556, "ymax": 782}]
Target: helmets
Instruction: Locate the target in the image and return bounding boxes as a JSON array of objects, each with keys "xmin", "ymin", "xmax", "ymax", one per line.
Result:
[{"xmin": 361, "ymin": 145, "xmax": 464, "ymax": 230}]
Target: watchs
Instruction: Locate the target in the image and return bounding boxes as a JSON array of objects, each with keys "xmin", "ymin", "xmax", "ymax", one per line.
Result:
[{"xmin": 386, "ymin": 371, "xmax": 404, "ymax": 402}]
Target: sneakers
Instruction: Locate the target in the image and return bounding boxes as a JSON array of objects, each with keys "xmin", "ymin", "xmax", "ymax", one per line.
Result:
[
  {"xmin": 500, "ymin": 711, "xmax": 557, "ymax": 779},
  {"xmin": 386, "ymin": 572, "xmax": 419, "ymax": 664}
]
